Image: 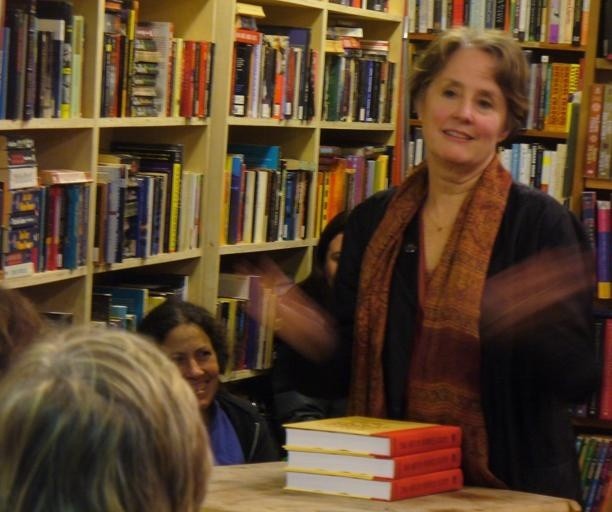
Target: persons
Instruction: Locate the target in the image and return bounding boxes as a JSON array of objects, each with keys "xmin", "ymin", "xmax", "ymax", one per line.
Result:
[
  {"xmin": 279, "ymin": 27, "xmax": 595, "ymax": 496},
  {"xmin": 0, "ymin": 285, "xmax": 277, "ymax": 512}
]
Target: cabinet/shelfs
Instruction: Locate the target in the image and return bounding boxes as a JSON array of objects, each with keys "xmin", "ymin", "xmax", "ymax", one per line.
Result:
[{"xmin": 0, "ymin": 0, "xmax": 611, "ymax": 433}]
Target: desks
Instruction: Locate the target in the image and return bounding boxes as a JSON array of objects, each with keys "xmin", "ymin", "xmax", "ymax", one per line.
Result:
[{"xmin": 194, "ymin": 462, "xmax": 578, "ymax": 510}]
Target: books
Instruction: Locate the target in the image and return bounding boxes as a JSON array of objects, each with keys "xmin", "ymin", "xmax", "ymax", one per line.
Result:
[
  {"xmin": 2, "ymin": 1, "xmax": 215, "ymax": 332},
  {"xmin": 215, "ymin": 0, "xmax": 397, "ymax": 374},
  {"xmin": 281, "ymin": 415, "xmax": 464, "ymax": 501},
  {"xmin": 574, "ymin": 319, "xmax": 612, "ymax": 512},
  {"xmin": 406, "ymin": 0, "xmax": 611, "ymax": 304}
]
